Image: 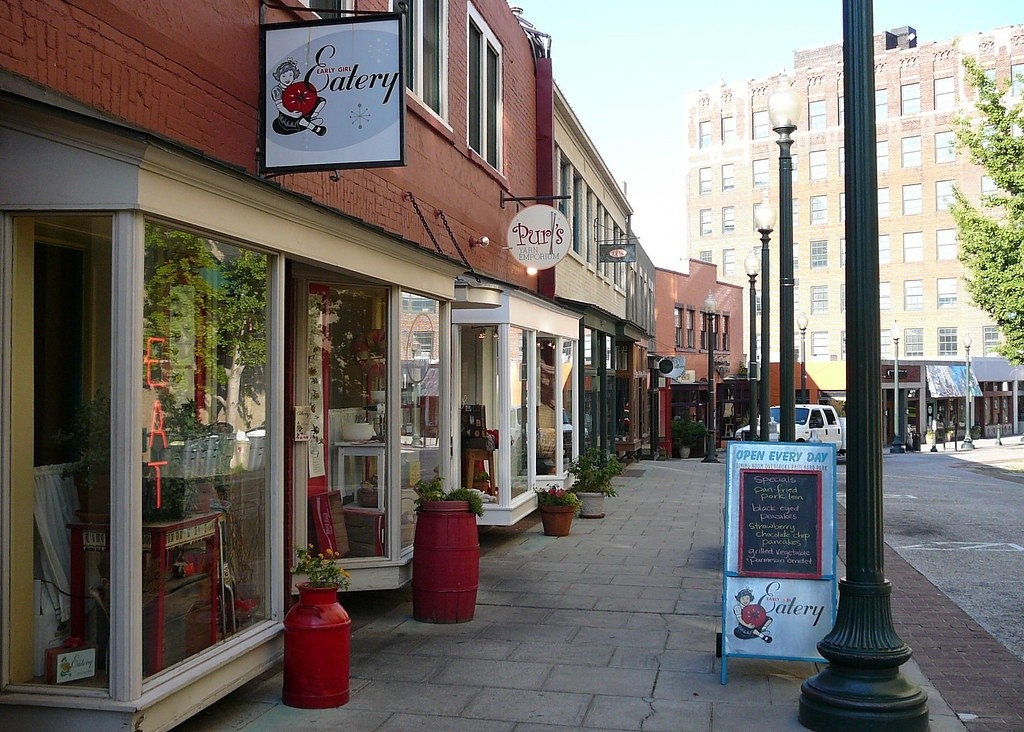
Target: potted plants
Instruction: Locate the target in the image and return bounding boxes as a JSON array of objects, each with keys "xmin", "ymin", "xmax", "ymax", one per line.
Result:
[
  {"xmin": 736, "ymin": 360, "xmax": 748, "ymax": 378},
  {"xmin": 970, "ymin": 426, "xmax": 981, "ymax": 440},
  {"xmin": 940, "ymin": 428, "xmax": 953, "ymax": 442},
  {"xmin": 566, "ymin": 446, "xmax": 623, "ymax": 519},
  {"xmin": 669, "ymin": 408, "xmax": 709, "ymax": 459},
  {"xmin": 475, "ymin": 470, "xmax": 489, "ymax": 494},
  {"xmin": 925, "ymin": 427, "xmax": 937, "ymax": 444}
]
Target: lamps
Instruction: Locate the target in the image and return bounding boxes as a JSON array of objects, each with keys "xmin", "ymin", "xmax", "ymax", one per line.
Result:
[
  {"xmin": 537, "ymin": 339, "xmax": 545, "ymax": 349},
  {"xmin": 478, "ymin": 327, "xmax": 487, "ymax": 339},
  {"xmin": 548, "ymin": 340, "xmax": 556, "ymax": 350},
  {"xmin": 492, "ymin": 325, "xmax": 499, "ymax": 338},
  {"xmin": 469, "ymin": 235, "xmax": 490, "ymax": 248}
]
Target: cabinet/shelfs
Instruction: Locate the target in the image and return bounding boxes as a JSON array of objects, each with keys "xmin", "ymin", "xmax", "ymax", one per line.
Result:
[{"xmin": 333, "ymin": 441, "xmax": 387, "ymax": 513}]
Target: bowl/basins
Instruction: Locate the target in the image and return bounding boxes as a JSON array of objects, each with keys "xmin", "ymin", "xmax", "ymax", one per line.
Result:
[
  {"xmin": 370, "ymin": 390, "xmax": 385, "ymax": 403},
  {"xmin": 343, "ymin": 422, "xmax": 373, "ymax": 442}
]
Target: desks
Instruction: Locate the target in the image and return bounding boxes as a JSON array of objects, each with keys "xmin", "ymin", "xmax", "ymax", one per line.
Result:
[
  {"xmin": 463, "ymin": 448, "xmax": 496, "ymax": 496},
  {"xmin": 64, "ymin": 511, "xmax": 223, "ymax": 678}
]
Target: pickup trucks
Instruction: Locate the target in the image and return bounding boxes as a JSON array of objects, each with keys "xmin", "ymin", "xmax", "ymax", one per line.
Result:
[{"xmin": 735, "ymin": 405, "xmax": 846, "ymax": 455}]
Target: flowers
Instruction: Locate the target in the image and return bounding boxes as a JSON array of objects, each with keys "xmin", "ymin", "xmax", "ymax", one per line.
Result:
[
  {"xmin": 290, "ymin": 542, "xmax": 352, "ymax": 591},
  {"xmin": 532, "ymin": 483, "xmax": 584, "ymax": 521},
  {"xmin": 413, "ymin": 474, "xmax": 484, "ymax": 518}
]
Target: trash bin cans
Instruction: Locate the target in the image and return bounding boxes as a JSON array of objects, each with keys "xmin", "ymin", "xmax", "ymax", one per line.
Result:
[{"xmin": 907, "ymin": 432, "xmax": 921, "ymax": 452}]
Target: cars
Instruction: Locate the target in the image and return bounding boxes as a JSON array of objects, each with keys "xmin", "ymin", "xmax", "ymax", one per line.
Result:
[{"xmin": 510, "ymin": 406, "xmax": 573, "ymax": 458}]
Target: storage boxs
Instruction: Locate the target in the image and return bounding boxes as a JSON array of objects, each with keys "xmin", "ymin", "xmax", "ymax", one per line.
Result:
[
  {"xmin": 311, "ymin": 489, "xmax": 351, "ymax": 559},
  {"xmin": 46, "ymin": 641, "xmax": 97, "ymax": 687},
  {"xmin": 88, "ymin": 573, "xmax": 211, "ymax": 672},
  {"xmin": 402, "ymin": 524, "xmax": 415, "ymax": 546}
]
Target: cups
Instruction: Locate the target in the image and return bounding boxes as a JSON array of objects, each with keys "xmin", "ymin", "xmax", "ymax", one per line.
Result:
[
  {"xmin": 615, "ymin": 438, "xmax": 619, "ymax": 441},
  {"xmin": 623, "ymin": 437, "xmax": 626, "ymax": 442}
]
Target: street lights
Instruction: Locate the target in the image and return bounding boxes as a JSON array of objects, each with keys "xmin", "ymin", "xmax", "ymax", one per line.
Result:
[
  {"xmin": 961, "ymin": 333, "xmax": 975, "ymax": 449},
  {"xmin": 700, "ymin": 289, "xmax": 722, "ymax": 464},
  {"xmin": 891, "ymin": 320, "xmax": 904, "ymax": 454},
  {"xmin": 745, "ymin": 254, "xmax": 760, "ymax": 442},
  {"xmin": 411, "ymin": 340, "xmax": 423, "ymax": 445},
  {"xmin": 798, "ymin": 312, "xmax": 808, "ymax": 404},
  {"xmin": 754, "ymin": 200, "xmax": 775, "ymax": 442},
  {"xmin": 767, "ymin": 81, "xmax": 802, "ymax": 442}
]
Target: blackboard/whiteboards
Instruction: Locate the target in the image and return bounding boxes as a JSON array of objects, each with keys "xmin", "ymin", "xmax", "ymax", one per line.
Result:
[{"xmin": 739, "ymin": 468, "xmax": 823, "ymax": 581}]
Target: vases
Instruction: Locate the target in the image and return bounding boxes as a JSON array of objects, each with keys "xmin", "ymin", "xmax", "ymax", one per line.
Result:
[
  {"xmin": 281, "ymin": 581, "xmax": 352, "ymax": 710},
  {"xmin": 411, "ymin": 500, "xmax": 480, "ymax": 624},
  {"xmin": 538, "ymin": 503, "xmax": 577, "ymax": 537}
]
